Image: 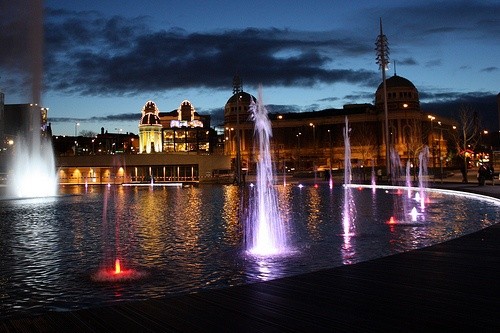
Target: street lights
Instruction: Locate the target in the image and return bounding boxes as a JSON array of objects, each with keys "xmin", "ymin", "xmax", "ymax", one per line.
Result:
[
  {"xmin": 232, "ymin": 72, "xmax": 244, "ymax": 186},
  {"xmin": 225, "ymin": 127, "xmax": 234, "ymax": 156},
  {"xmin": 373, "ymin": 16, "xmax": 391, "ymax": 185},
  {"xmin": 309, "ymin": 122, "xmax": 315, "ymax": 171},
  {"xmin": 296, "ymin": 133, "xmax": 302, "ymax": 171},
  {"xmin": 427, "ymin": 115, "xmax": 436, "ymax": 167},
  {"xmin": 403, "ymin": 103, "xmax": 410, "ymax": 158}
]
